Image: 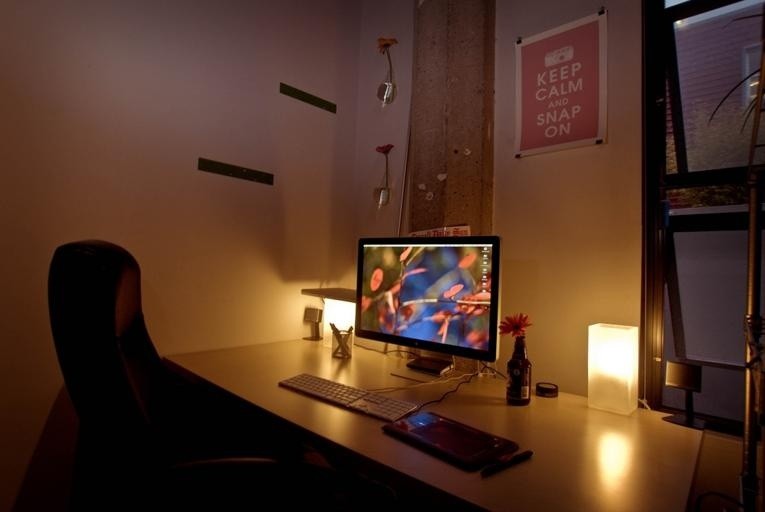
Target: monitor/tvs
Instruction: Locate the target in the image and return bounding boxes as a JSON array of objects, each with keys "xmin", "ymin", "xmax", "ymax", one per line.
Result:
[{"xmin": 355, "ymin": 236, "xmax": 502, "ymax": 383}]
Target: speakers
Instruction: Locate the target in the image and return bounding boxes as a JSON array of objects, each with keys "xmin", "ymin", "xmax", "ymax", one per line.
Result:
[{"xmin": 302, "ymin": 307, "xmax": 323, "ymax": 341}]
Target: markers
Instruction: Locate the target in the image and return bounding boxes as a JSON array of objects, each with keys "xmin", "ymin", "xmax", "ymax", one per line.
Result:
[{"xmin": 481, "ymin": 451, "xmax": 532, "ymax": 478}]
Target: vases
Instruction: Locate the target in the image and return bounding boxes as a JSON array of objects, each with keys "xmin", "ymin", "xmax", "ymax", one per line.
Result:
[
  {"xmin": 377, "ymin": 81, "xmax": 397, "ymax": 103},
  {"xmin": 373, "ymin": 185, "xmax": 389, "ymax": 206}
]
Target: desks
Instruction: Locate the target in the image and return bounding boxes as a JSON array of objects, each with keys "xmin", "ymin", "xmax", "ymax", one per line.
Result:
[{"xmin": 161, "ymin": 334, "xmax": 706, "ymax": 512}]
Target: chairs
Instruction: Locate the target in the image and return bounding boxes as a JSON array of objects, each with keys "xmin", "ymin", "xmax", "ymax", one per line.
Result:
[{"xmin": 48, "ymin": 239, "xmax": 398, "ymax": 511}]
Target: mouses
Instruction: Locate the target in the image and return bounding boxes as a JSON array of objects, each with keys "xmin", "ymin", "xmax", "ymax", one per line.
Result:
[{"xmin": 461, "ymin": 431, "xmax": 494, "ymax": 454}]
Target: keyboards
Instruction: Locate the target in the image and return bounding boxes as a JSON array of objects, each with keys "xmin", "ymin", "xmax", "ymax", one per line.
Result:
[{"xmin": 279, "ymin": 374, "xmax": 417, "ymax": 420}]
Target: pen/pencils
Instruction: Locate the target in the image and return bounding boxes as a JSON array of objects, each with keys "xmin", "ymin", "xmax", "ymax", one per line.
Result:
[{"xmin": 329, "ymin": 322, "xmax": 353, "ymax": 358}]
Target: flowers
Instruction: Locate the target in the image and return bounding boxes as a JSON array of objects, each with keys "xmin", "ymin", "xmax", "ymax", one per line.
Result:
[
  {"xmin": 376, "ymin": 37, "xmax": 398, "ymax": 83},
  {"xmin": 498, "ymin": 312, "xmax": 533, "ymax": 337},
  {"xmin": 376, "ymin": 144, "xmax": 394, "ymax": 188}
]
